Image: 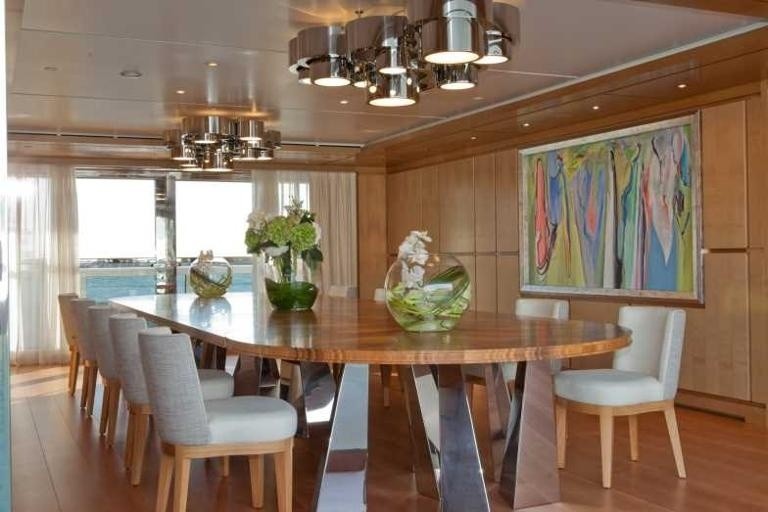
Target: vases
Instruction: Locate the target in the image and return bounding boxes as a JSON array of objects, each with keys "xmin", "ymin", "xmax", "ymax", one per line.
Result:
[
  {"xmin": 385, "ymin": 255, "xmax": 472, "ymax": 332},
  {"xmin": 188, "ymin": 257, "xmax": 233, "ymax": 298},
  {"xmin": 264, "ymin": 256, "xmax": 319, "ymax": 313}
]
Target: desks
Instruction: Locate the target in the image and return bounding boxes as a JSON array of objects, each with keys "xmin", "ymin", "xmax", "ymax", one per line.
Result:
[{"xmin": 104, "ymin": 287, "xmax": 635, "ymax": 510}]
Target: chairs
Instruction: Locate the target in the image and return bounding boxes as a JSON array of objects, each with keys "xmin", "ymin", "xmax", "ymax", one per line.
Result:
[
  {"xmin": 545, "ymin": 303, "xmax": 685, "ymax": 490},
  {"xmin": 134, "ymin": 324, "xmax": 303, "ymax": 511},
  {"xmin": 59, "ymin": 288, "xmax": 236, "ymax": 487}
]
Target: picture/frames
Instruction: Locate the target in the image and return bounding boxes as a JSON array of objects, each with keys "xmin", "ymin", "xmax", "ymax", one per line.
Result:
[{"xmin": 519, "ymin": 107, "xmax": 704, "ymax": 309}]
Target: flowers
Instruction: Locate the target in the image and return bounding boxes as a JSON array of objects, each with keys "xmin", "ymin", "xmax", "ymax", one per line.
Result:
[
  {"xmin": 244, "ymin": 195, "xmax": 324, "ymax": 283},
  {"xmin": 385, "ymin": 230, "xmax": 472, "ymax": 329}
]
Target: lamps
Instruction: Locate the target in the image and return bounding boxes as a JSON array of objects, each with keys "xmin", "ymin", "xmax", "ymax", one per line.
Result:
[
  {"xmin": 288, "ymin": 0, "xmax": 512, "ymax": 107},
  {"xmin": 164, "ymin": 115, "xmax": 281, "ymax": 170}
]
